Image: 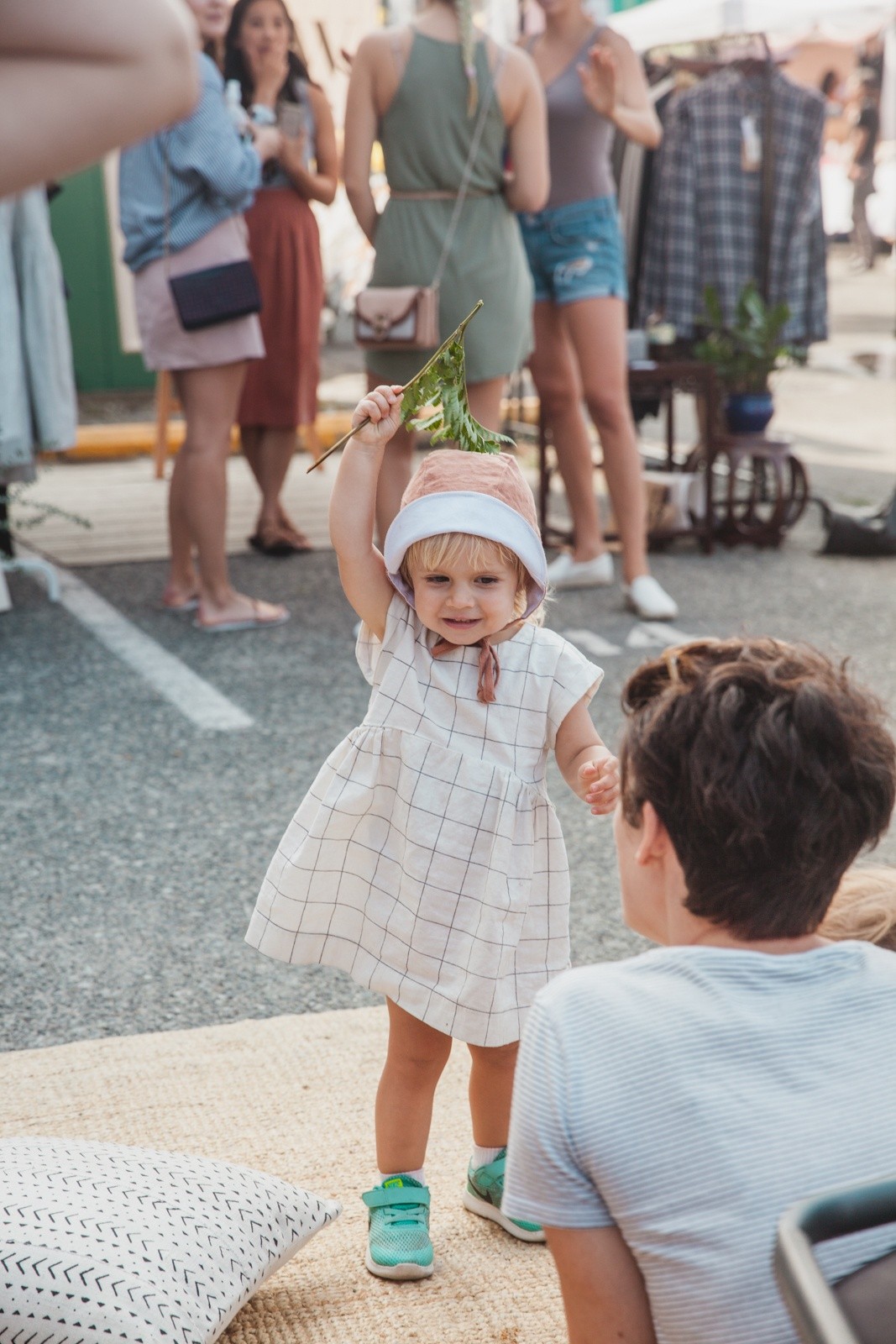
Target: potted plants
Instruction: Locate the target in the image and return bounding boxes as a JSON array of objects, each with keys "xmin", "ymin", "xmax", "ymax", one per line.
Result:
[{"xmin": 694, "ymin": 279, "xmax": 798, "ymax": 435}]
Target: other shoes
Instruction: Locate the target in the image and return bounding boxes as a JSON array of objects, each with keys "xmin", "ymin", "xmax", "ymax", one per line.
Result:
[
  {"xmin": 277, "ymin": 526, "xmax": 312, "ymax": 553},
  {"xmin": 247, "ymin": 521, "xmax": 294, "ymax": 558}
]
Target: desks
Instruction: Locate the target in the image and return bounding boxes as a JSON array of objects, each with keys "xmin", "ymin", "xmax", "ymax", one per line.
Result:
[{"xmin": 501, "ymin": 359, "xmax": 716, "ymax": 556}]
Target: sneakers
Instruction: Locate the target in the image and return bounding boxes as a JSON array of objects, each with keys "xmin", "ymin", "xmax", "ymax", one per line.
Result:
[
  {"xmin": 464, "ymin": 1147, "xmax": 547, "ymax": 1243},
  {"xmin": 618, "ymin": 575, "xmax": 681, "ymax": 619},
  {"xmin": 544, "ymin": 552, "xmax": 616, "ymax": 589},
  {"xmin": 361, "ymin": 1174, "xmax": 435, "ymax": 1278}
]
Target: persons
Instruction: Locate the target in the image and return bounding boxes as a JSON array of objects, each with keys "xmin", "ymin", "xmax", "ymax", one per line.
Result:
[
  {"xmin": 0, "ymin": 3, "xmax": 209, "ymax": 203},
  {"xmin": 494, "ymin": 640, "xmax": 894, "ymax": 1344},
  {"xmin": 497, "ymin": 0, "xmax": 675, "ymax": 622},
  {"xmin": 338, "ymin": 0, "xmax": 551, "ymax": 557},
  {"xmin": 118, "ymin": 1, "xmax": 293, "ymax": 640},
  {"xmin": 329, "ymin": 378, "xmax": 629, "ymax": 1286},
  {"xmin": 196, "ymin": 1, "xmax": 338, "ymax": 561}
]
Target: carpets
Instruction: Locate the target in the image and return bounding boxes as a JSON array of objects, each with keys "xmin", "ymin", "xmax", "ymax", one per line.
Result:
[{"xmin": 1, "ymin": 1005, "xmax": 573, "ymax": 1343}]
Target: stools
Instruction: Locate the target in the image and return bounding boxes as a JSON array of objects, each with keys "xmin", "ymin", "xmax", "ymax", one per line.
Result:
[{"xmin": 680, "ymin": 438, "xmax": 810, "ymax": 550}]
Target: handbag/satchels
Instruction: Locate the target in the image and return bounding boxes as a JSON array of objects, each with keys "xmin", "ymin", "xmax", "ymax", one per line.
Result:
[
  {"xmin": 170, "ymin": 260, "xmax": 261, "ymax": 329},
  {"xmin": 354, "ymin": 283, "xmax": 441, "ymax": 351}
]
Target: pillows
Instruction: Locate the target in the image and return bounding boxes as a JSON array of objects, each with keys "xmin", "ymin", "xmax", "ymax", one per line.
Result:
[{"xmin": 1, "ymin": 1136, "xmax": 346, "ymax": 1343}]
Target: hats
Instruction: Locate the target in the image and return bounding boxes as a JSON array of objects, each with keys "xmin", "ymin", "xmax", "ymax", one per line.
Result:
[{"xmin": 383, "ymin": 448, "xmax": 546, "ymax": 704}]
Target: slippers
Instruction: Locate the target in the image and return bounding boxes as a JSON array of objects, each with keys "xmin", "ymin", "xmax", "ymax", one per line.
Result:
[
  {"xmin": 171, "ymin": 592, "xmax": 200, "ymax": 617},
  {"xmin": 191, "ymin": 593, "xmax": 291, "ymax": 633}
]
